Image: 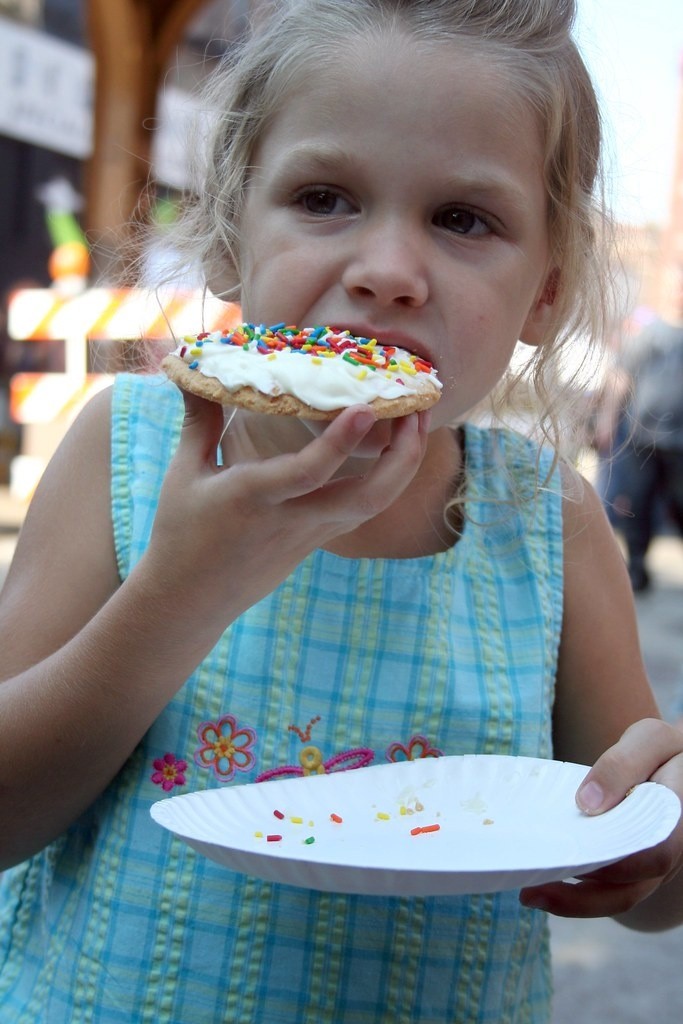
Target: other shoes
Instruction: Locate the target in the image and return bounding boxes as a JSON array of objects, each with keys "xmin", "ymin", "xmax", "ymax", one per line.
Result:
[{"xmin": 628, "ymin": 562, "xmax": 649, "ymax": 592}]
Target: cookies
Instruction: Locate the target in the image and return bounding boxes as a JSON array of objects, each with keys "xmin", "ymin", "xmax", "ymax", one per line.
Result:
[{"xmin": 160, "ymin": 321, "xmax": 442, "ymax": 423}]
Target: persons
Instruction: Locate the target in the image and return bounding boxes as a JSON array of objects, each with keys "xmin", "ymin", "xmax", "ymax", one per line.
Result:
[
  {"xmin": 586, "ymin": 323, "xmax": 683, "ymax": 594},
  {"xmin": 0, "ymin": 0, "xmax": 683, "ymax": 1024}
]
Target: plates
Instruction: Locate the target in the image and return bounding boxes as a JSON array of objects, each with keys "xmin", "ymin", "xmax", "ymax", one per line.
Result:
[{"xmin": 147, "ymin": 753, "xmax": 682, "ymax": 898}]
